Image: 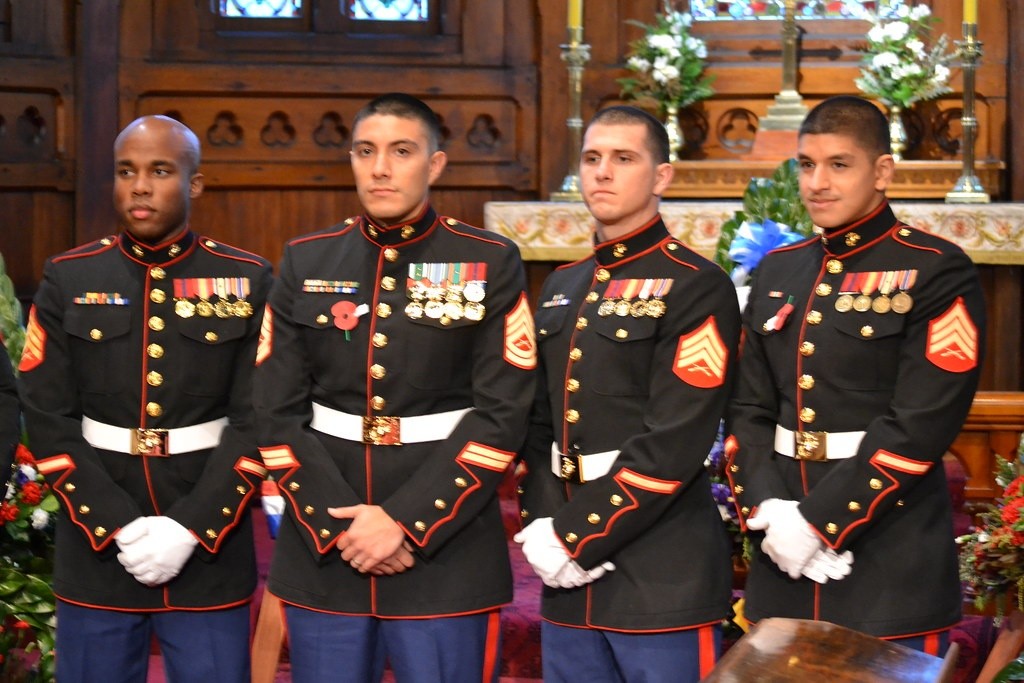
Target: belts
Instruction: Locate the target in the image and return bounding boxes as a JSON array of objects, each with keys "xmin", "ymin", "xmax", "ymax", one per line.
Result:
[
  {"xmin": 308, "ymin": 399, "xmax": 477, "ymax": 446},
  {"xmin": 550, "ymin": 441, "xmax": 626, "ymax": 485},
  {"xmin": 80, "ymin": 414, "xmax": 232, "ymax": 458},
  {"xmin": 773, "ymin": 424, "xmax": 868, "ymax": 463}
]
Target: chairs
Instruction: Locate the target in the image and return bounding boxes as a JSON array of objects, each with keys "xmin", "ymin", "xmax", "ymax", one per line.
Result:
[{"xmin": 702, "ymin": 578, "xmax": 1024, "ymax": 683}]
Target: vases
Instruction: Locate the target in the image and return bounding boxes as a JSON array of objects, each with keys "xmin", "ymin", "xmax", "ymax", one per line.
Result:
[
  {"xmin": 663, "ymin": 109, "xmax": 686, "ymax": 162},
  {"xmin": 889, "ymin": 106, "xmax": 908, "ymax": 161}
]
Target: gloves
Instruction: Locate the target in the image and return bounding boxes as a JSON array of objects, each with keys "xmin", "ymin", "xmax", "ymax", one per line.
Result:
[
  {"xmin": 744, "ymin": 498, "xmax": 856, "ymax": 584},
  {"xmin": 513, "ymin": 516, "xmax": 617, "ymax": 589},
  {"xmin": 113, "ymin": 513, "xmax": 200, "ymax": 587}
]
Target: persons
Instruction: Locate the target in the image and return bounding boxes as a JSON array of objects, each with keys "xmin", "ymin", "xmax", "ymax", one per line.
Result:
[
  {"xmin": 20, "ymin": 115, "xmax": 274, "ymax": 683},
  {"xmin": 723, "ymin": 97, "xmax": 988, "ymax": 659},
  {"xmin": 0, "ymin": 338, "xmax": 21, "ymax": 490},
  {"xmin": 514, "ymin": 104, "xmax": 743, "ymax": 683},
  {"xmin": 252, "ymin": 92, "xmax": 538, "ymax": 683}
]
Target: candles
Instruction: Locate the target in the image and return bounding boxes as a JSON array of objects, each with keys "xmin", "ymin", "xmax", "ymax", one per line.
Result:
[
  {"xmin": 962, "ymin": 0, "xmax": 977, "ymax": 26},
  {"xmin": 568, "ymin": 0, "xmax": 582, "ymax": 28}
]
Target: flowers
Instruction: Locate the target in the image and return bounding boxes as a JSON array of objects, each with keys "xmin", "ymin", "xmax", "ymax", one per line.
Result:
[
  {"xmin": 853, "ymin": 2, "xmax": 983, "ymax": 108},
  {"xmin": 0, "ymin": 458, "xmax": 60, "ymax": 683},
  {"xmin": 955, "ymin": 432, "xmax": 1024, "ymax": 629},
  {"xmin": 614, "ymin": 0, "xmax": 717, "ymax": 110}
]
